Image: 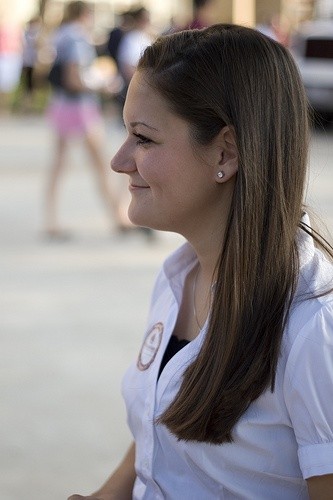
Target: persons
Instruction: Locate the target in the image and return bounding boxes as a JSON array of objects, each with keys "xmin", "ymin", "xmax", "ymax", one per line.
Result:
[
  {"xmin": 33, "ymin": 1, "xmax": 154, "ymax": 241},
  {"xmin": 14, "ymin": 1, "xmax": 212, "ymax": 110},
  {"xmin": 63, "ymin": 24, "xmax": 333, "ymax": 500}
]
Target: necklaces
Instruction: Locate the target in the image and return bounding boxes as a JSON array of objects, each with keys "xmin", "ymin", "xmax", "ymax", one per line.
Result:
[{"xmin": 192, "ymin": 264, "xmax": 216, "ymax": 331}]
